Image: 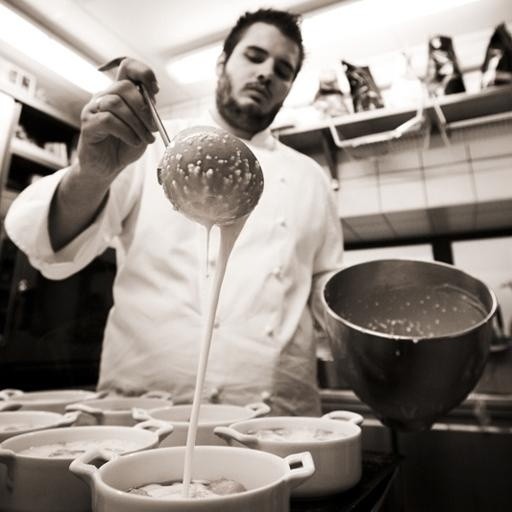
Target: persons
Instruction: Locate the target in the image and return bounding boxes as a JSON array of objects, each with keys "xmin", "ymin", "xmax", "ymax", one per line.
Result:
[{"xmin": 3, "ymin": 6, "xmax": 343, "ymax": 416}]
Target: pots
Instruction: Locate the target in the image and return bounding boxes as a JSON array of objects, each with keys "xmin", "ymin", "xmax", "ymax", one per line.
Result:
[{"xmin": 318, "ymin": 254, "xmax": 502, "ymax": 436}]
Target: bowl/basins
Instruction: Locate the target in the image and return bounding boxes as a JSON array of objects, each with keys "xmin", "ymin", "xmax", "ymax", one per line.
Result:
[
  {"xmin": 0, "ymin": 410, "xmax": 83, "ymax": 441},
  {"xmin": 130, "ymin": 401, "xmax": 271, "ymax": 447},
  {"xmin": 0, "ymin": 386, "xmax": 110, "ymax": 414},
  {"xmin": 68, "ymin": 445, "xmax": 317, "ymax": 512},
  {"xmin": 0, "ymin": 418, "xmax": 175, "ymax": 511},
  {"xmin": 62, "ymin": 388, "xmax": 175, "ymax": 427},
  {"xmin": 211, "ymin": 409, "xmax": 366, "ymax": 497}
]
